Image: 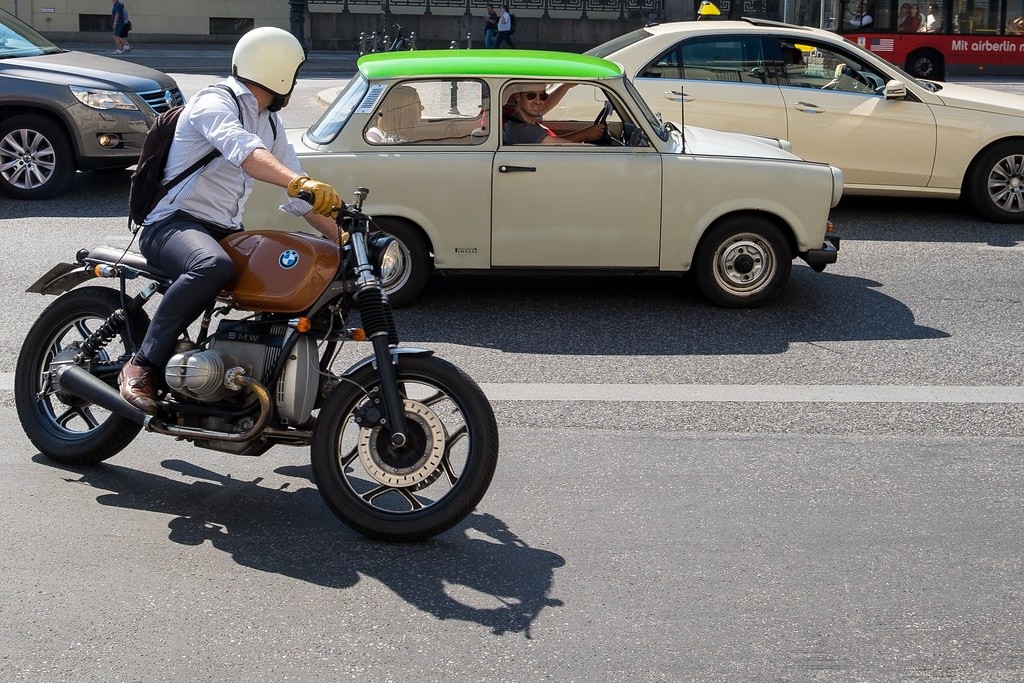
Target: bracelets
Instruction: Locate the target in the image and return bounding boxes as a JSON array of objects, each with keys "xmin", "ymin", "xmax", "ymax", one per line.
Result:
[{"xmin": 114, "ymin": 23, "xmax": 116, "ymax": 24}]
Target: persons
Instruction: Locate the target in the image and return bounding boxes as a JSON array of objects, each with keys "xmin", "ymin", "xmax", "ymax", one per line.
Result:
[
  {"xmin": 484, "ymin": 3, "xmax": 516, "ymax": 49},
  {"xmin": 501, "ymin": 80, "xmax": 605, "ymax": 145},
  {"xmin": 843, "ymin": 3, "xmax": 875, "ymax": 30},
  {"xmin": 897, "ymin": 3, "xmax": 943, "ymax": 33},
  {"xmin": 781, "ymin": 45, "xmax": 839, "ymax": 89},
  {"xmin": 116, "ymin": 23, "xmax": 350, "ymax": 416},
  {"xmin": 110, "ymin": 0, "xmax": 135, "ymax": 54}
]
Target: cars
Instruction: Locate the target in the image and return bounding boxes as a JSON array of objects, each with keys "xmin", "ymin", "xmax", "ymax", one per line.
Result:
[
  {"xmin": 235, "ymin": 48, "xmax": 848, "ymax": 309},
  {"xmin": 0, "ymin": 5, "xmax": 187, "ymax": 202},
  {"xmin": 535, "ymin": 15, "xmax": 1024, "ymax": 225}
]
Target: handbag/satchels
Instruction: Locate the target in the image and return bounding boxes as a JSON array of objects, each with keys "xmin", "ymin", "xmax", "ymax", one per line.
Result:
[{"xmin": 128, "ymin": 20, "xmax": 132, "ymax": 30}]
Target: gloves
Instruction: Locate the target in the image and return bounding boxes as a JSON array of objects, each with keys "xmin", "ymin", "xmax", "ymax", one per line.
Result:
[
  {"xmin": 337, "ymin": 232, "xmax": 351, "ymax": 246},
  {"xmin": 286, "ymin": 174, "xmax": 341, "ymax": 220}
]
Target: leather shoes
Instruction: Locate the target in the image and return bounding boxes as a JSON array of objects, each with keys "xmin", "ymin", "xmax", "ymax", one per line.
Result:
[{"xmin": 117, "ymin": 356, "xmax": 159, "ymax": 415}]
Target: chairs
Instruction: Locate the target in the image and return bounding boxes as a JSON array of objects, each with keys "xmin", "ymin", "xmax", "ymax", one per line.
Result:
[
  {"xmin": 379, "ymin": 86, "xmax": 424, "ymax": 140},
  {"xmin": 916, "ymin": 14, "xmax": 926, "ymax": 32},
  {"xmin": 782, "ymin": 45, "xmax": 800, "ymax": 86}
]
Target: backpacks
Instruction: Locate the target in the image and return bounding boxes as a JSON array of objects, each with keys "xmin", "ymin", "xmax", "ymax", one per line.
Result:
[
  {"xmin": 128, "ymin": 83, "xmax": 278, "ymax": 225},
  {"xmin": 508, "ymin": 13, "xmax": 516, "ymax": 35}
]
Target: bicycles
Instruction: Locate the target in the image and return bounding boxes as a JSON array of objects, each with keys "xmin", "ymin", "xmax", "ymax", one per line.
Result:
[{"xmin": 353, "ymin": 9, "xmax": 421, "ymax": 56}]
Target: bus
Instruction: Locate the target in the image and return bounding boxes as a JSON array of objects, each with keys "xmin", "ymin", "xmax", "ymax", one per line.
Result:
[{"xmin": 784, "ymin": 0, "xmax": 1024, "ymax": 84}]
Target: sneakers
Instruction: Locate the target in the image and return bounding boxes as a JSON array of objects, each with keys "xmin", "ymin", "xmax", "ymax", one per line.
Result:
[{"xmin": 112, "ymin": 46, "xmax": 135, "ymax": 55}]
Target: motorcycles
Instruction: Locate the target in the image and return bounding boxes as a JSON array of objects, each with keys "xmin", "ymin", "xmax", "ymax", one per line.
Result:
[{"xmin": 11, "ymin": 185, "xmax": 501, "ymax": 544}]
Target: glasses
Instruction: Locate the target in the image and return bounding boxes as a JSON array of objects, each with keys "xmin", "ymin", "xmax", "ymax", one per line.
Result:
[
  {"xmin": 901, "ymin": 6, "xmax": 909, "ymax": 9},
  {"xmin": 520, "ymin": 91, "xmax": 548, "ymax": 102},
  {"xmin": 911, "ymin": 7, "xmax": 918, "ymax": 10}
]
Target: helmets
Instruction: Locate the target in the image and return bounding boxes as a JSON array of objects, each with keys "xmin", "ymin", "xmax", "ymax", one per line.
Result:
[{"xmin": 231, "ymin": 27, "xmax": 308, "ymax": 95}]
